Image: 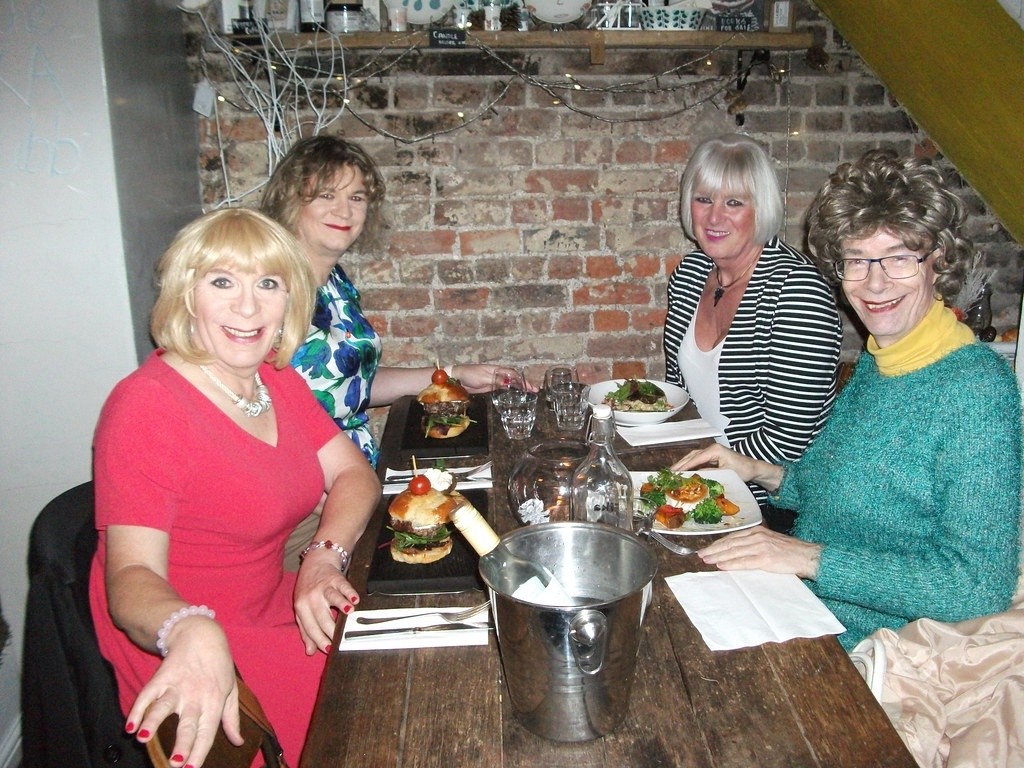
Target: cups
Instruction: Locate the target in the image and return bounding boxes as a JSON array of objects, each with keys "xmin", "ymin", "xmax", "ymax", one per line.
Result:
[
  {"xmin": 491, "ymin": 366, "xmax": 527, "ymax": 412},
  {"xmin": 545, "ymin": 364, "xmax": 581, "ymax": 412},
  {"xmin": 498, "ymin": 392, "xmax": 539, "ymax": 439},
  {"xmin": 508, "ymin": 438, "xmax": 589, "ymax": 523},
  {"xmin": 619, "ymin": 496, "xmax": 656, "ymax": 530},
  {"xmin": 550, "ymin": 383, "xmax": 589, "ymax": 432}
]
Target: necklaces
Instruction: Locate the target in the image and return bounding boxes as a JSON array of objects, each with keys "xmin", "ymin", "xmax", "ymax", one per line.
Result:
[
  {"xmin": 714, "ymin": 252, "xmax": 761, "ymax": 307},
  {"xmin": 200, "ymin": 364, "xmax": 272, "ymax": 416}
]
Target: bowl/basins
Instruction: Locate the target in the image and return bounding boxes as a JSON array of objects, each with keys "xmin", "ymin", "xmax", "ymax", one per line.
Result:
[{"xmin": 584, "ymin": 378, "xmax": 688, "ymax": 426}]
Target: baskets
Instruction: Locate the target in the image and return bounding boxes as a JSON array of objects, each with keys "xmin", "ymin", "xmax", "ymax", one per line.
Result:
[{"xmin": 638, "ymin": 5, "xmax": 707, "ymax": 31}]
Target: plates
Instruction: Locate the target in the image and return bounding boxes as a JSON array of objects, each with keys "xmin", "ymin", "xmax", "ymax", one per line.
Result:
[
  {"xmin": 366, "ymin": 490, "xmax": 490, "ymax": 595},
  {"xmin": 618, "ymin": 469, "xmax": 763, "ymax": 553},
  {"xmin": 402, "ymin": 396, "xmax": 491, "ymax": 455}
]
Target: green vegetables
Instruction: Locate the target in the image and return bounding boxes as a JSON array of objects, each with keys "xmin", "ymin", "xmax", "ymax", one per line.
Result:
[
  {"xmin": 603, "ymin": 375, "xmax": 665, "ymax": 409},
  {"xmin": 647, "ymin": 466, "xmax": 695, "ymax": 493}
]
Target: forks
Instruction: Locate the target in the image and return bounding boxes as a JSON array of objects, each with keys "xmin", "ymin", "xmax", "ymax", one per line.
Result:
[
  {"xmin": 387, "ymin": 462, "xmax": 494, "ymax": 476},
  {"xmin": 650, "ymin": 531, "xmax": 694, "ymax": 555},
  {"xmin": 357, "ymin": 601, "xmax": 490, "ymax": 622}
]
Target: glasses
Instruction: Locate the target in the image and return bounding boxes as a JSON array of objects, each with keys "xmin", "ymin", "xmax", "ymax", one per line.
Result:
[{"xmin": 833, "ymin": 243, "xmax": 941, "ymax": 282}]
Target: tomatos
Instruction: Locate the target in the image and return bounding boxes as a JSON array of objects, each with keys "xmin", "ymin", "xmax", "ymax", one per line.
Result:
[
  {"xmin": 432, "ymin": 370, "xmax": 448, "ymax": 384},
  {"xmin": 409, "ymin": 474, "xmax": 431, "ymax": 495}
]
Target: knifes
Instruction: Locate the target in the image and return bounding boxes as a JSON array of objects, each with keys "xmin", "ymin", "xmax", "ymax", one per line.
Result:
[
  {"xmin": 384, "ymin": 477, "xmax": 495, "ymax": 486},
  {"xmin": 345, "ymin": 624, "xmax": 494, "ymax": 638}
]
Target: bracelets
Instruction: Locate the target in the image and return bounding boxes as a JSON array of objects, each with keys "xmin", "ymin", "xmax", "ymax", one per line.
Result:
[
  {"xmin": 443, "ymin": 363, "xmax": 457, "ymax": 379},
  {"xmin": 298, "ymin": 539, "xmax": 349, "ymax": 571},
  {"xmin": 157, "ymin": 603, "xmax": 217, "ymax": 656}
]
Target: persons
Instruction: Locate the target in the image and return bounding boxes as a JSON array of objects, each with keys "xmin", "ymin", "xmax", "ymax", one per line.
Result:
[
  {"xmin": 258, "ymin": 135, "xmax": 540, "ymax": 469},
  {"xmin": 663, "ymin": 145, "xmax": 1024, "ymax": 655},
  {"xmin": 661, "ymin": 136, "xmax": 846, "ymax": 530},
  {"xmin": 87, "ymin": 205, "xmax": 383, "ymax": 768}
]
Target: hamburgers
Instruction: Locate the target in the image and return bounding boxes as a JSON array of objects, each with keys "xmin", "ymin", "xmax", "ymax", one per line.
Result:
[
  {"xmin": 388, "ymin": 487, "xmax": 458, "ymax": 564},
  {"xmin": 416, "ymin": 380, "xmax": 477, "ymax": 439}
]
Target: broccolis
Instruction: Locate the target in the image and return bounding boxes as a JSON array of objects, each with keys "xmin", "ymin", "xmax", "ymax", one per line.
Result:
[
  {"xmin": 685, "ymin": 498, "xmax": 722, "ymax": 524},
  {"xmin": 641, "ymin": 491, "xmax": 667, "ymax": 509},
  {"xmin": 699, "ymin": 477, "xmax": 724, "ymax": 497}
]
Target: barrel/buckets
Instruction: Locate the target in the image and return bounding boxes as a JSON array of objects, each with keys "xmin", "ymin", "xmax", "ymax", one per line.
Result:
[{"xmin": 477, "ymin": 524, "xmax": 658, "ymax": 740}]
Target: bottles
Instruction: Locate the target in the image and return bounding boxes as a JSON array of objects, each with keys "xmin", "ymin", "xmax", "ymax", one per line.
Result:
[
  {"xmin": 451, "ymin": 498, "xmax": 578, "ymax": 609},
  {"xmin": 573, "ymin": 405, "xmax": 635, "ymax": 528}
]
